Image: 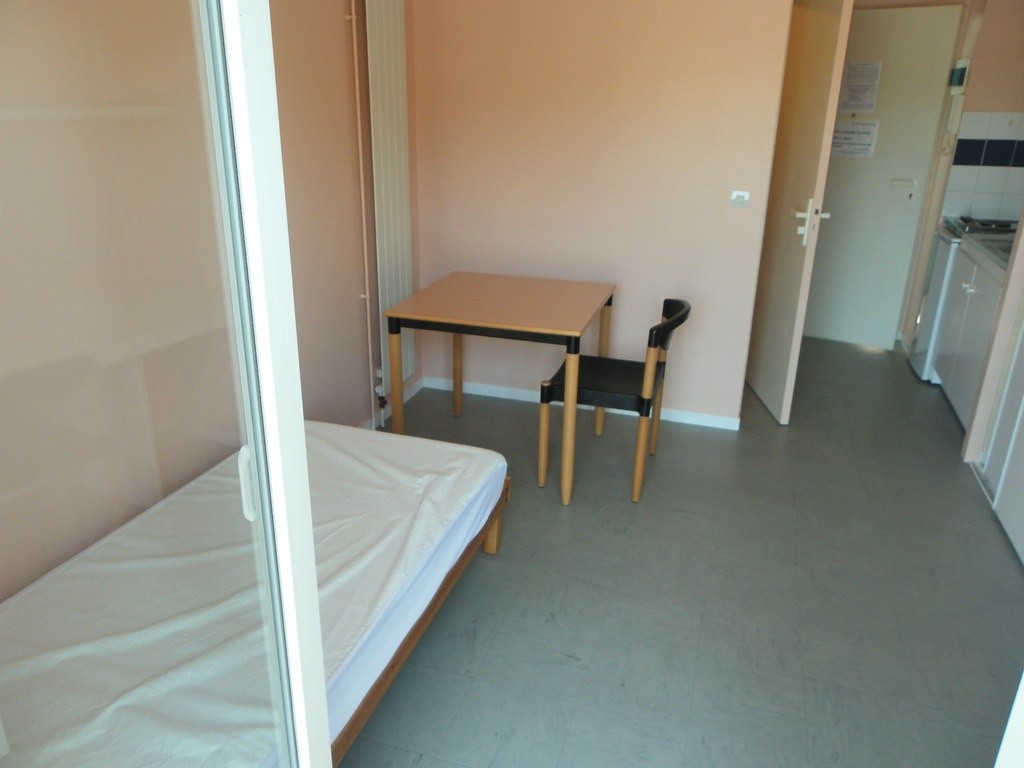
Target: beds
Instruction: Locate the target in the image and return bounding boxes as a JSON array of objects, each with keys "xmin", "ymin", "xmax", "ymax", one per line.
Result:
[{"xmin": 0, "ymin": 414, "xmax": 509, "ymax": 767}]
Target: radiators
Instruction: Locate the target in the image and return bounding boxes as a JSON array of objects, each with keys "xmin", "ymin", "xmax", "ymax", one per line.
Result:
[{"xmin": 365, "ymin": 0, "xmax": 417, "ymax": 396}]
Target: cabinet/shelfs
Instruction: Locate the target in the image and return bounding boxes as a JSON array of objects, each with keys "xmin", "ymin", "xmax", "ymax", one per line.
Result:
[{"xmin": 933, "ymin": 248, "xmax": 1004, "ymax": 432}]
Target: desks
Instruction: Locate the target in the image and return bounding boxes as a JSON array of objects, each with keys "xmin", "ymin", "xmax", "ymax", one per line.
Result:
[{"xmin": 385, "ymin": 272, "xmax": 618, "ymax": 506}]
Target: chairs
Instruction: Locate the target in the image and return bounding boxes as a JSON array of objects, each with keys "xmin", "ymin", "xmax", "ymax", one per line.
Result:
[{"xmin": 535, "ymin": 299, "xmax": 695, "ymax": 501}]
